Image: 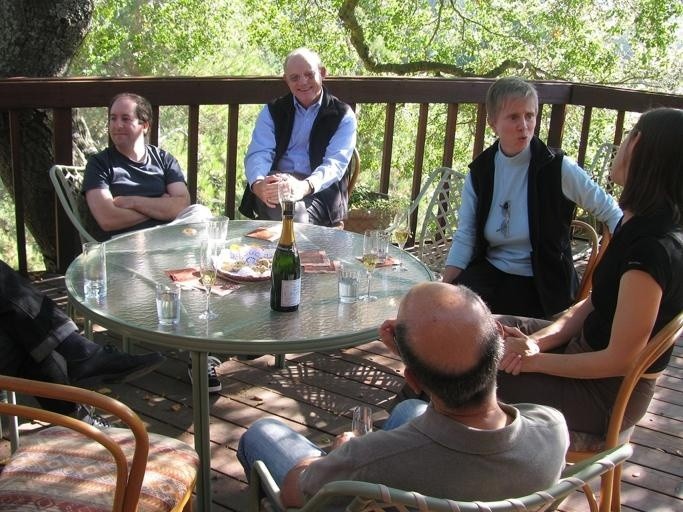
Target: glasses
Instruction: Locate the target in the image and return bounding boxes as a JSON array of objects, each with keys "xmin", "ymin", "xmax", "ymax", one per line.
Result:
[
  {"xmin": 288, "ymin": 71, "xmax": 314, "ymax": 82},
  {"xmin": 496, "ymin": 200, "xmax": 512, "ymax": 239}
]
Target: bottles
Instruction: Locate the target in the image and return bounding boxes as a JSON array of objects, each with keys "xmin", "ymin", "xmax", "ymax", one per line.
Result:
[{"xmin": 269, "ymin": 199, "xmax": 301, "ymax": 313}]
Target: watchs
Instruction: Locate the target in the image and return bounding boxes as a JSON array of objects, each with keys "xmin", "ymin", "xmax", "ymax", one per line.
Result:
[{"xmin": 307, "ymin": 180, "xmax": 314, "ymax": 196}]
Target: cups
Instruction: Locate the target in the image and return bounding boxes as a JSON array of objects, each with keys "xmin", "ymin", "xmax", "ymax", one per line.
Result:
[
  {"xmin": 294, "ymin": 201, "xmax": 311, "ymax": 231},
  {"xmin": 81, "ymin": 240, "xmax": 107, "ymax": 301},
  {"xmin": 336, "ymin": 265, "xmax": 360, "ymax": 305},
  {"xmin": 375, "ymin": 230, "xmax": 391, "ymax": 265},
  {"xmin": 351, "ymin": 406, "xmax": 373, "ymax": 438},
  {"xmin": 155, "ymin": 281, "xmax": 182, "ymax": 326}
]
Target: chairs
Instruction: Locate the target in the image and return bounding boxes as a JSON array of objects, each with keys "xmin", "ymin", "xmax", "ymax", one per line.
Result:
[
  {"xmin": 49, "ymin": 165, "xmax": 132, "ymax": 364},
  {"xmin": 1, "ymin": 376, "xmax": 199, "ymax": 512},
  {"xmin": 322, "ymin": 147, "xmax": 359, "ymax": 230},
  {"xmin": 568, "ymin": 220, "xmax": 596, "ymax": 302},
  {"xmin": 383, "ymin": 167, "xmax": 467, "ymax": 285},
  {"xmin": 504, "ymin": 236, "xmax": 683, "ymax": 512},
  {"xmin": 248, "ymin": 442, "xmax": 633, "ymax": 512}
]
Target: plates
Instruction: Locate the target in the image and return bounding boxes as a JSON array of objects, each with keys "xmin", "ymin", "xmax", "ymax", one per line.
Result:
[
  {"xmin": 213, "ymin": 268, "xmax": 268, "ymax": 285},
  {"xmin": 210, "ymin": 248, "xmax": 274, "ymax": 286}
]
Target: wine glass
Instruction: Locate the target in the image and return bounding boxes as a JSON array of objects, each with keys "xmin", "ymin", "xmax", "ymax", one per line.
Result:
[
  {"xmin": 358, "ymin": 227, "xmax": 380, "ymax": 302},
  {"xmin": 198, "ymin": 237, "xmax": 220, "ymax": 322},
  {"xmin": 206, "ymin": 214, "xmax": 229, "ymax": 281},
  {"xmin": 392, "ymin": 206, "xmax": 411, "ymax": 275},
  {"xmin": 278, "ymin": 173, "xmax": 290, "ymax": 222}
]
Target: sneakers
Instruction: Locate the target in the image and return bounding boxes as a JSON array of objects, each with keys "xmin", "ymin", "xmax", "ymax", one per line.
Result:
[{"xmin": 188, "ymin": 351, "xmax": 222, "ymax": 392}]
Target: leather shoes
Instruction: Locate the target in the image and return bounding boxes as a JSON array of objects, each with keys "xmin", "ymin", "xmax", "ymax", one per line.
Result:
[
  {"xmin": 72, "ymin": 402, "xmax": 111, "ymax": 432},
  {"xmin": 67, "ymin": 342, "xmax": 167, "ymax": 389}
]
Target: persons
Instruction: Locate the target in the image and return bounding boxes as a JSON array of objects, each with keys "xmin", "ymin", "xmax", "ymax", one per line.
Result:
[
  {"xmin": 235, "ymin": 282, "xmax": 572, "ymax": 511},
  {"xmin": 0, "ymin": 260, "xmax": 166, "ymax": 430},
  {"xmin": 79, "ymin": 92, "xmax": 261, "ymax": 393},
  {"xmin": 238, "ymin": 46, "xmax": 358, "ymax": 360},
  {"xmin": 441, "ymin": 77, "xmax": 624, "ymax": 320},
  {"xmin": 385, "ymin": 106, "xmax": 683, "ymax": 436}
]
